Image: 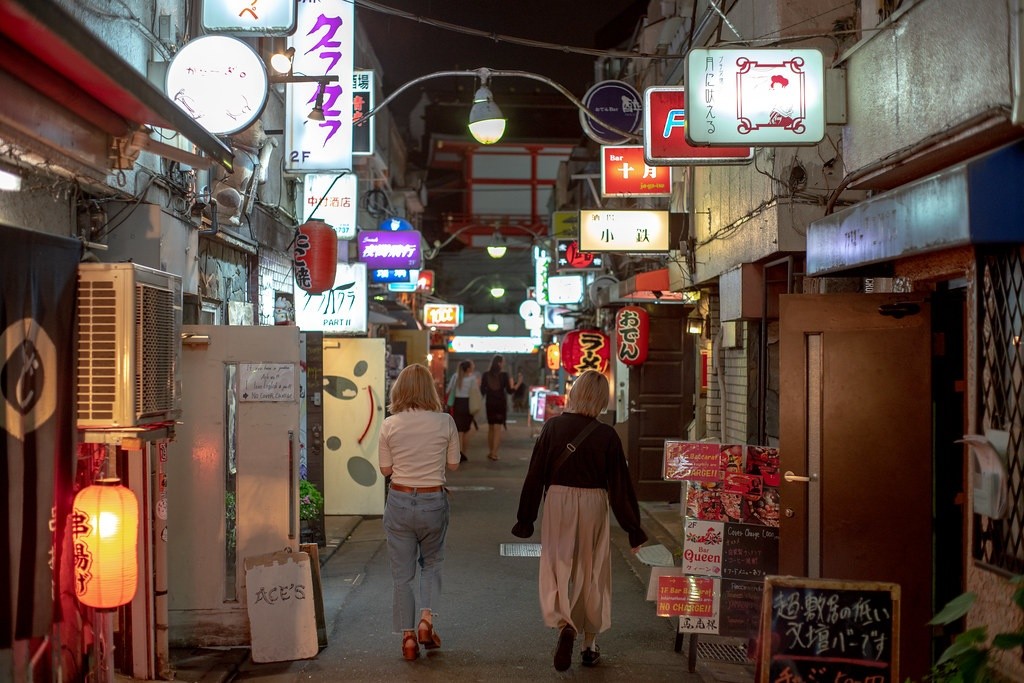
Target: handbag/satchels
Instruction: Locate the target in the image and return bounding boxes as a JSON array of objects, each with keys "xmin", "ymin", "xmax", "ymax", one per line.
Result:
[
  {"xmin": 468, "ymin": 383, "xmax": 483, "ymax": 415},
  {"xmin": 447, "ymin": 376, "xmax": 457, "ymax": 406}
]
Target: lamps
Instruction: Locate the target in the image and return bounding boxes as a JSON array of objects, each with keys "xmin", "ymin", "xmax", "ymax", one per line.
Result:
[
  {"xmin": 684, "ymin": 304, "xmax": 711, "ymax": 338},
  {"xmin": 487, "ymin": 317, "xmax": 500, "ymax": 332},
  {"xmin": 271, "ymin": 47, "xmax": 295, "ymax": 77},
  {"xmin": 491, "ymin": 273, "xmax": 505, "ymax": 298},
  {"xmin": 487, "ymin": 221, "xmax": 507, "ymax": 259},
  {"xmin": 468, "ymin": 67, "xmax": 508, "ymax": 146},
  {"xmin": 307, "ymin": 81, "xmax": 330, "ymax": 121}
]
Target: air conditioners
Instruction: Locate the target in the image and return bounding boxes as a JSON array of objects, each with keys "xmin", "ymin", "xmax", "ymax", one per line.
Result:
[{"xmin": 75, "ymin": 261, "xmax": 184, "ymax": 430}]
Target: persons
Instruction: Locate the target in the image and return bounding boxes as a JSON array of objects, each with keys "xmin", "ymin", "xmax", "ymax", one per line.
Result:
[
  {"xmin": 446, "ymin": 359, "xmax": 481, "ymax": 462},
  {"xmin": 378, "ymin": 363, "xmax": 461, "ymax": 659},
  {"xmin": 510, "ymin": 370, "xmax": 647, "ymax": 671},
  {"xmin": 479, "ymin": 353, "xmax": 524, "ymax": 460}
]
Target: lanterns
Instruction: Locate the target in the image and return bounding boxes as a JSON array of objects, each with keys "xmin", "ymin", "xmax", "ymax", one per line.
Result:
[
  {"xmin": 562, "ymin": 325, "xmax": 610, "ymax": 376},
  {"xmin": 293, "ymin": 216, "xmax": 337, "ymax": 296},
  {"xmin": 72, "ymin": 476, "xmax": 137, "ymax": 614},
  {"xmin": 615, "ymin": 306, "xmax": 649, "ymax": 369},
  {"xmin": 547, "ymin": 345, "xmax": 560, "ymax": 370}
]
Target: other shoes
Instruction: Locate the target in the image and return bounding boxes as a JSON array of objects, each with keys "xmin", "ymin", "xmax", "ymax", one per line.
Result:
[
  {"xmin": 554, "ymin": 623, "xmax": 577, "ymax": 672},
  {"xmin": 487, "ymin": 453, "xmax": 498, "ymax": 461},
  {"xmin": 580, "ymin": 644, "xmax": 601, "ymax": 667},
  {"xmin": 460, "ymin": 452, "xmax": 467, "ymax": 462}
]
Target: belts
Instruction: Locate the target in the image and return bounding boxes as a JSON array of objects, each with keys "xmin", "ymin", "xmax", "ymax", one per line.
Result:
[{"xmin": 390, "ymin": 484, "xmax": 446, "ymax": 493}]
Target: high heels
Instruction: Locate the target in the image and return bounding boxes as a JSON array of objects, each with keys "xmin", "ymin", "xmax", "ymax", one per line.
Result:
[
  {"xmin": 417, "ymin": 619, "xmax": 442, "ymax": 650},
  {"xmin": 402, "ymin": 635, "xmax": 419, "ymax": 660}
]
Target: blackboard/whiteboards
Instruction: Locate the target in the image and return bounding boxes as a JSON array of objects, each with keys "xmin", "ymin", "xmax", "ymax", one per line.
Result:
[{"xmin": 755, "ymin": 575, "xmax": 902, "ymax": 683}]
